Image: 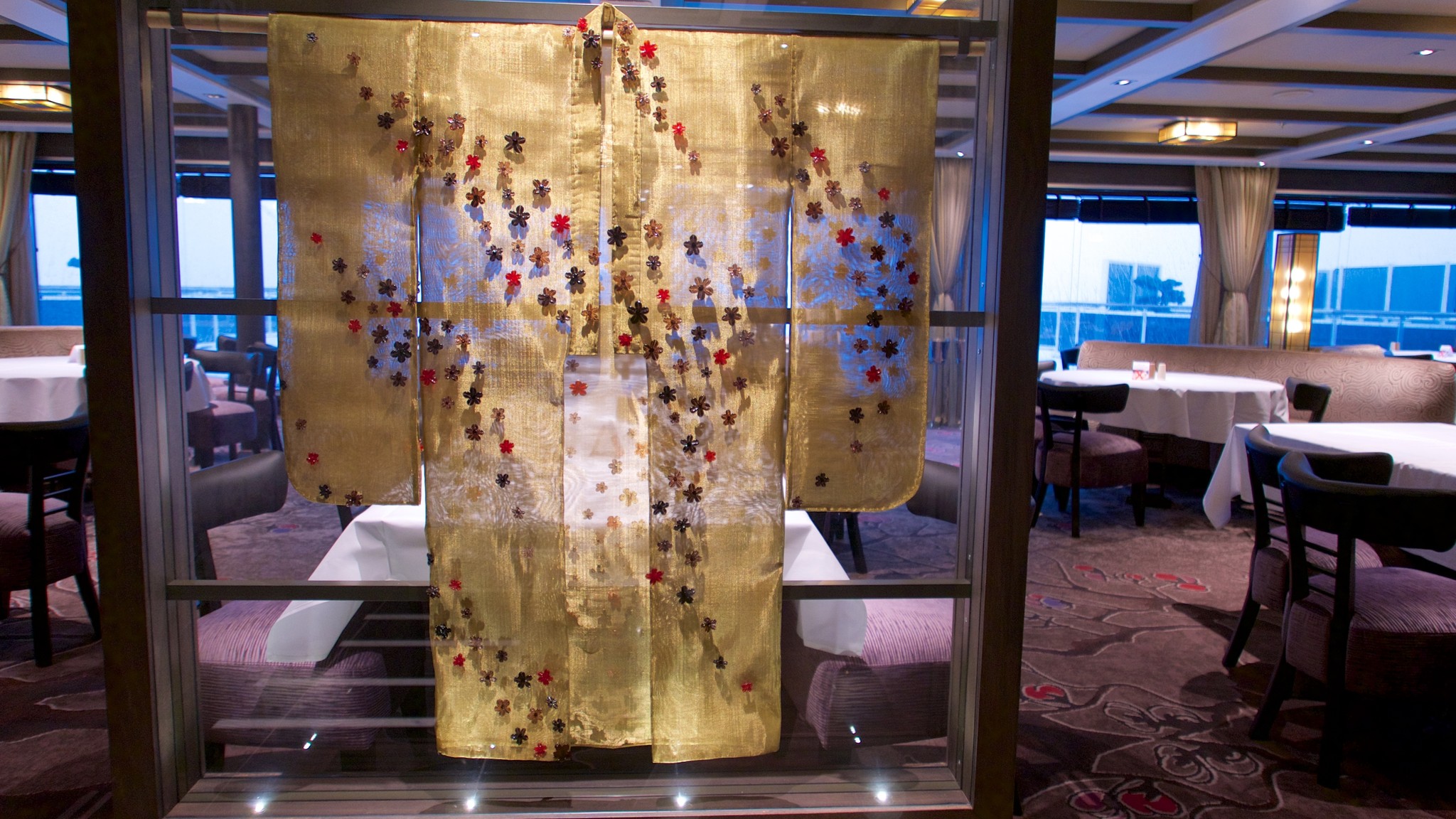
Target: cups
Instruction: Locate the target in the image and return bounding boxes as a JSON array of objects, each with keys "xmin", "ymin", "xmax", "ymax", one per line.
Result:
[
  {"xmin": 1390, "ymin": 342, "xmax": 1400, "ymax": 351},
  {"xmin": 1157, "ymin": 363, "xmax": 1166, "ymax": 380},
  {"xmin": 1149, "ymin": 362, "xmax": 1156, "ymax": 379},
  {"xmin": 76, "ymin": 348, "xmax": 85, "ymax": 365}
]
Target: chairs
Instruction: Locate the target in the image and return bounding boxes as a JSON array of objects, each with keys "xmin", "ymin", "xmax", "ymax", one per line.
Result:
[
  {"xmin": 184, "ymin": 334, "xmax": 283, "ymax": 467},
  {"xmin": 189, "ymin": 448, "xmax": 423, "ymax": 774},
  {"xmin": 1246, "ymin": 451, "xmax": 1456, "ymax": 799},
  {"xmin": 1284, "ymin": 377, "xmax": 1332, "ymax": 423},
  {"xmin": 780, "ymin": 457, "xmax": 958, "ymax": 751},
  {"xmin": 0, "ymin": 423, "xmax": 100, "ymax": 671},
  {"xmin": 1031, "ymin": 359, "xmax": 1148, "ymax": 539},
  {"xmin": 1222, "ymin": 425, "xmax": 1395, "ymax": 697}
]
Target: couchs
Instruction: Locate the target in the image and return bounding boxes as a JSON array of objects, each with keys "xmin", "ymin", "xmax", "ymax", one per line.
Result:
[{"xmin": 1074, "ymin": 340, "xmax": 1456, "ymax": 472}]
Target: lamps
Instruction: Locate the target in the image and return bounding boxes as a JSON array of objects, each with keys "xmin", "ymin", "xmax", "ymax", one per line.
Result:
[
  {"xmin": 0, "ymin": 82, "xmax": 72, "ymax": 113},
  {"xmin": 1159, "ymin": 122, "xmax": 1237, "ymax": 146},
  {"xmin": 1266, "ymin": 233, "xmax": 1320, "ymax": 351}
]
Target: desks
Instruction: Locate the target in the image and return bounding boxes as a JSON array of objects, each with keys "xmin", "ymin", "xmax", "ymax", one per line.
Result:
[
  {"xmin": 264, "ymin": 375, "xmax": 874, "ymax": 735},
  {"xmin": 1202, "ymin": 421, "xmax": 1456, "ymax": 573},
  {"xmin": 0, "ymin": 354, "xmax": 216, "ymax": 492},
  {"xmin": 1038, "ymin": 369, "xmax": 1291, "ymax": 510}
]
